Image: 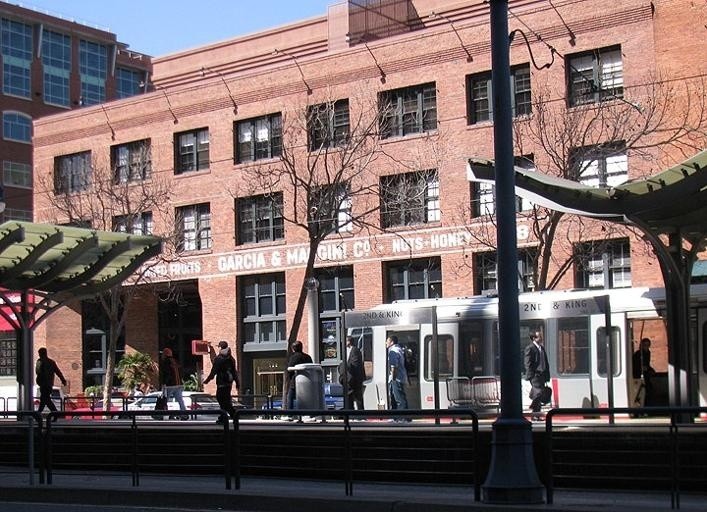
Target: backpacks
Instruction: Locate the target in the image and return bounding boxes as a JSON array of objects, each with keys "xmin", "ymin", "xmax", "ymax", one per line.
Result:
[{"xmin": 401, "ymin": 343, "xmax": 419, "ymax": 374}]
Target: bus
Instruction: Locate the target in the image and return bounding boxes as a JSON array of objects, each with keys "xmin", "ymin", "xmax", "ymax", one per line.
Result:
[{"xmin": 346, "ymin": 282, "xmax": 706, "ymax": 424}]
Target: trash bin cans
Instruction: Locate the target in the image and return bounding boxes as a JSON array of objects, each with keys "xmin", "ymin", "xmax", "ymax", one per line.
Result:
[{"xmin": 295, "ymin": 362, "xmax": 324, "ymax": 409}]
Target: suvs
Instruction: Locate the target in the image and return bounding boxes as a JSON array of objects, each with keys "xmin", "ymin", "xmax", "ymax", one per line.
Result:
[{"xmin": 261, "ymin": 383, "xmax": 344, "ymax": 410}]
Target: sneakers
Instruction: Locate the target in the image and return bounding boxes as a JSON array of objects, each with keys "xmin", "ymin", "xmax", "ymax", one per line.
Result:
[
  {"xmin": 150, "ymin": 414, "xmax": 165, "ymax": 421},
  {"xmin": 279, "ymin": 415, "xmax": 293, "ymax": 422},
  {"xmin": 176, "ymin": 414, "xmax": 189, "ymax": 420},
  {"xmin": 531, "ymin": 415, "xmax": 544, "ymax": 422},
  {"xmin": 302, "ymin": 416, "xmax": 317, "ymax": 423}
]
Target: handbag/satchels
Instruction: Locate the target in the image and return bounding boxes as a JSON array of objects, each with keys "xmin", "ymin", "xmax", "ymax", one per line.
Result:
[
  {"xmin": 526, "ymin": 386, "xmax": 553, "ymax": 404},
  {"xmin": 155, "ymin": 394, "xmax": 168, "ymax": 412}
]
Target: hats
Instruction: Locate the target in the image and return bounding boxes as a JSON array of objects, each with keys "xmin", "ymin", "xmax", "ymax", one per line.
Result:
[
  {"xmin": 215, "ymin": 340, "xmax": 228, "ymax": 348},
  {"xmin": 159, "ymin": 347, "xmax": 173, "ymax": 355}
]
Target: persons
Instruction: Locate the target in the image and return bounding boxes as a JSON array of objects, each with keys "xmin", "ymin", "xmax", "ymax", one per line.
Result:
[
  {"xmin": 630, "ymin": 338, "xmax": 651, "ymax": 418},
  {"xmin": 111, "ymin": 386, "xmax": 155, "ymax": 402},
  {"xmin": 33, "ymin": 348, "xmax": 67, "ymax": 421},
  {"xmin": 386, "ymin": 334, "xmax": 412, "ymax": 423},
  {"xmin": 524, "ymin": 331, "xmax": 550, "ymax": 420},
  {"xmin": 346, "ymin": 336, "xmax": 367, "ymax": 421},
  {"xmin": 150, "ymin": 347, "xmax": 189, "ymax": 421},
  {"xmin": 280, "ymin": 341, "xmax": 313, "ymax": 420},
  {"xmin": 89, "ymin": 389, "xmax": 104, "ymax": 397},
  {"xmin": 201, "ymin": 341, "xmax": 240, "ymax": 423},
  {"xmin": 186, "ymin": 401, "xmax": 202, "ymax": 421}
]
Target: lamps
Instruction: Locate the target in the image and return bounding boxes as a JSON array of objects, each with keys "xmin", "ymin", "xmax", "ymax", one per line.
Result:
[
  {"xmin": 139, "ymin": 81, "xmax": 179, "ymax": 124},
  {"xmin": 200, "ymin": 71, "xmax": 238, "ymax": 116},
  {"xmin": 271, "ymin": 48, "xmax": 312, "ymax": 96},
  {"xmin": 346, "ymin": 36, "xmax": 387, "ymax": 84},
  {"xmin": 549, "ymin": 0, "xmax": 575, "ymax": 47},
  {"xmin": 428, "ymin": 11, "xmax": 473, "ymax": 63},
  {"xmin": 78, "ymin": 95, "xmax": 116, "ymax": 137}
]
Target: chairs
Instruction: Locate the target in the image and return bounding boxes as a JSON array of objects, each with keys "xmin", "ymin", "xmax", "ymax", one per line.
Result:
[
  {"xmin": 445, "ymin": 376, "xmax": 473, "ymax": 424},
  {"xmin": 469, "ymin": 374, "xmax": 500, "ymax": 424}
]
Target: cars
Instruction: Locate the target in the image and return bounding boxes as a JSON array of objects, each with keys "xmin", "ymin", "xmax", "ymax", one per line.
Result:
[{"xmin": 0, "ymin": 385, "xmax": 247, "ymax": 421}]
